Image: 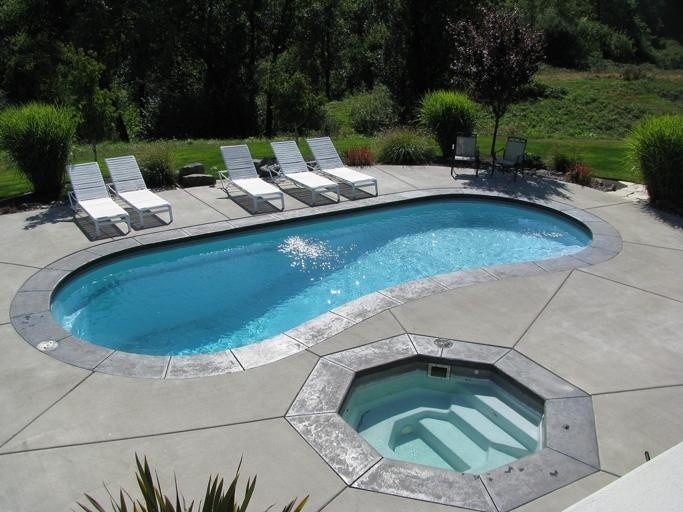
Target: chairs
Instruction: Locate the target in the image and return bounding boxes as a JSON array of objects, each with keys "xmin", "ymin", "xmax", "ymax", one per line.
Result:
[
  {"xmin": 449, "ymin": 132, "xmax": 528, "ymax": 183},
  {"xmin": 64, "ymin": 154, "xmax": 173, "ymax": 240},
  {"xmin": 218, "ymin": 134, "xmax": 377, "ymax": 213}
]
